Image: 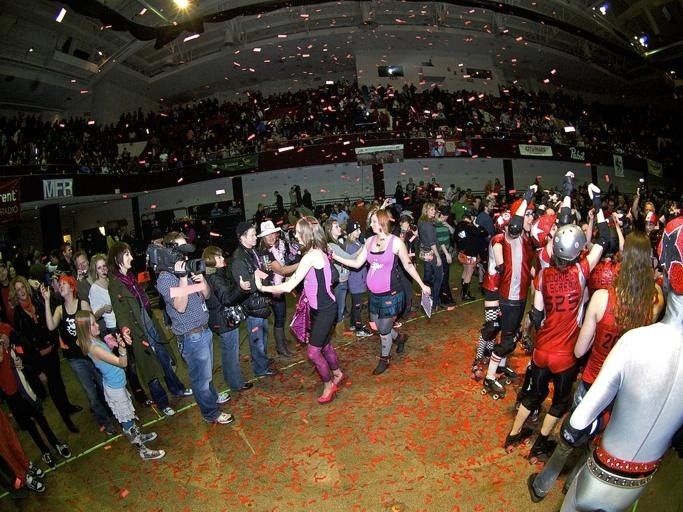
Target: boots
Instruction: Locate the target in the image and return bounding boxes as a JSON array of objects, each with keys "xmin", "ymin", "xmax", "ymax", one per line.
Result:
[
  {"xmin": 333, "ymin": 320, "xmax": 352, "ymax": 346},
  {"xmin": 283, "ymin": 332, "xmax": 291, "ymax": 345},
  {"xmin": 124, "ymin": 424, "xmax": 165, "ymax": 460},
  {"xmin": 461, "ymin": 283, "xmax": 475, "ymax": 301},
  {"xmin": 134, "ymin": 422, "xmax": 157, "ymax": 443},
  {"xmin": 274, "ymin": 327, "xmax": 294, "ymax": 357}
]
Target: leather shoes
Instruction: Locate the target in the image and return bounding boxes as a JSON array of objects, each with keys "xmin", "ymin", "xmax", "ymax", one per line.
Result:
[
  {"xmin": 141, "ymin": 400, "xmax": 152, "ymax": 406},
  {"xmin": 69, "ymin": 405, "xmax": 83, "ymax": 411},
  {"xmin": 68, "ymin": 423, "xmax": 80, "ymax": 433},
  {"xmin": 240, "ymin": 383, "xmax": 253, "ymax": 390}
]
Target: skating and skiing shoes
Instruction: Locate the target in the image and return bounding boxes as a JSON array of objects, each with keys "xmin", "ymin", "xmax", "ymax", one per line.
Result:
[
  {"xmin": 481, "ymin": 376, "xmax": 505, "ymax": 400},
  {"xmin": 526, "ymin": 441, "xmax": 557, "ymax": 465},
  {"xmin": 496, "ymin": 365, "xmax": 516, "ymax": 384},
  {"xmin": 505, "ymin": 428, "xmax": 534, "ymax": 454},
  {"xmin": 472, "ymin": 361, "xmax": 484, "ymax": 379}
]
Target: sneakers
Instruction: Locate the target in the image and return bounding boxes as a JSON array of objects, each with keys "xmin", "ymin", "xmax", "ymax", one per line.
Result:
[
  {"xmin": 56, "ymin": 441, "xmax": 71, "ymax": 458},
  {"xmin": 163, "ymin": 407, "xmax": 175, "ymax": 416},
  {"xmin": 254, "ymin": 368, "xmax": 278, "ymax": 376},
  {"xmin": 393, "ymin": 334, "xmax": 408, "ymax": 353},
  {"xmin": 373, "ymin": 357, "xmax": 390, "ymax": 374},
  {"xmin": 26, "ymin": 474, "xmax": 45, "ymax": 492},
  {"xmin": 184, "ymin": 388, "xmax": 193, "ymax": 395},
  {"xmin": 29, "ymin": 465, "xmax": 44, "ymax": 478},
  {"xmin": 203, "ymin": 412, "xmax": 233, "ymax": 424},
  {"xmin": 356, "ymin": 328, "xmax": 374, "ymax": 337},
  {"xmin": 528, "ymin": 472, "xmax": 544, "ymax": 503},
  {"xmin": 105, "ymin": 427, "xmax": 117, "ymax": 435},
  {"xmin": 42, "ymin": 453, "xmax": 56, "ymax": 470},
  {"xmin": 216, "ymin": 392, "xmax": 231, "ymax": 403},
  {"xmin": 350, "ymin": 326, "xmax": 356, "ymax": 331},
  {"xmin": 393, "ymin": 320, "xmax": 402, "ymax": 328}
]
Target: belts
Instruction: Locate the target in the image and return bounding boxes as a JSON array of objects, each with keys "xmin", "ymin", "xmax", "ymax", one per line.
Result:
[{"xmin": 189, "ymin": 324, "xmax": 208, "ymax": 335}]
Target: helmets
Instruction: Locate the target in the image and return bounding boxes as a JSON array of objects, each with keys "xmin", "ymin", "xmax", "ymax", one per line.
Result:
[
  {"xmin": 658, "ymin": 216, "xmax": 683, "ymax": 295},
  {"xmin": 553, "ymin": 224, "xmax": 587, "ymax": 260},
  {"xmin": 511, "ymin": 198, "xmax": 535, "ymax": 217},
  {"xmin": 530, "ymin": 214, "xmax": 557, "ymax": 247},
  {"xmin": 596, "ymin": 228, "xmax": 619, "ymax": 253},
  {"xmin": 496, "ymin": 212, "xmax": 512, "ymax": 228}
]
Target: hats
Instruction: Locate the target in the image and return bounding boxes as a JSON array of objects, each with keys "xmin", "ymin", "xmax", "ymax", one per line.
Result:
[
  {"xmin": 257, "ymin": 220, "xmax": 281, "ymax": 237},
  {"xmin": 346, "ymin": 219, "xmax": 360, "ymax": 234},
  {"xmin": 236, "ymin": 222, "xmax": 252, "ymax": 239}
]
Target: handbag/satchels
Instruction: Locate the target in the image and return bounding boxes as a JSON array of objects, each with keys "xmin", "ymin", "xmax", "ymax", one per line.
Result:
[
  {"xmin": 419, "ymin": 249, "xmax": 433, "ymax": 262},
  {"xmin": 458, "ymin": 250, "xmax": 467, "ymax": 264},
  {"xmin": 223, "ymin": 303, "xmax": 248, "ymax": 327}
]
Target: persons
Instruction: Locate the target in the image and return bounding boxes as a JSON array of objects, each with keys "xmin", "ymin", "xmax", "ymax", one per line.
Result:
[
  {"xmin": 471, "ymin": 170, "xmax": 683, "ymax": 512},
  {"xmin": 2, "ymin": 176, "xmax": 505, "ymax": 496},
  {"xmin": 2, "ymin": 79, "xmax": 683, "ymax": 201}
]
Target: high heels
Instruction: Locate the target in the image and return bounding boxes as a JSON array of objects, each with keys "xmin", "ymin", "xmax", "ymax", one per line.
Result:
[
  {"xmin": 318, "ymin": 384, "xmax": 337, "ymax": 403},
  {"xmin": 333, "ymin": 372, "xmax": 346, "ymax": 386}
]
